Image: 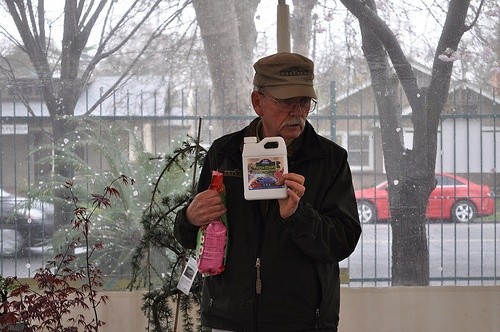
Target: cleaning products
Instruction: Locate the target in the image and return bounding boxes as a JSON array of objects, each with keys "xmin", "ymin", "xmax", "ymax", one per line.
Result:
[{"xmin": 243, "ymin": 137, "xmax": 289, "ymax": 201}]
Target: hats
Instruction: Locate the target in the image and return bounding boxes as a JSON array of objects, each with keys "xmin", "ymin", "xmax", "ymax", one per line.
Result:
[{"xmin": 253, "ymin": 52, "xmax": 318, "ymax": 100}]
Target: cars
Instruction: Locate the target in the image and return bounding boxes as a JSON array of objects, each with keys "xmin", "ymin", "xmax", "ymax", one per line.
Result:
[
  {"xmin": 0, "ymin": 189, "xmax": 55, "ymax": 258},
  {"xmin": 354, "ymin": 173, "xmax": 496, "ymax": 224}
]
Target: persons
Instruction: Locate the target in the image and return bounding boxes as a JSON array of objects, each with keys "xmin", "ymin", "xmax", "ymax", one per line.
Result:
[{"xmin": 173, "ymin": 51, "xmax": 361, "ymax": 332}]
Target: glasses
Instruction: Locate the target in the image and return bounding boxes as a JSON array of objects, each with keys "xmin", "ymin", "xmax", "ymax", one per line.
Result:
[{"xmin": 263, "ymin": 95, "xmax": 316, "ymax": 113}]
